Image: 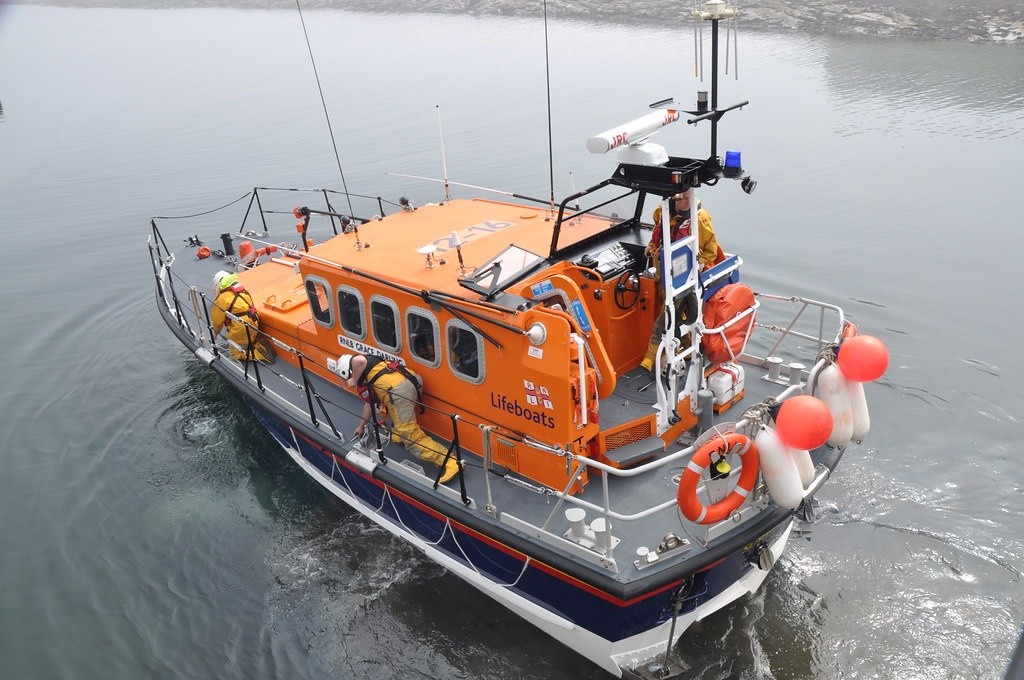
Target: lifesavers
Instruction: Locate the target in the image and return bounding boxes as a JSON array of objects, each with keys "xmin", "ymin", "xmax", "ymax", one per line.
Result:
[{"xmin": 679, "ymin": 433, "xmax": 760, "ymax": 524}]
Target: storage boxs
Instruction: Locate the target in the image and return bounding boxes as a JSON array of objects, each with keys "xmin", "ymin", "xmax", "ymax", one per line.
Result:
[{"xmin": 707, "ymin": 364, "xmax": 745, "ymax": 406}]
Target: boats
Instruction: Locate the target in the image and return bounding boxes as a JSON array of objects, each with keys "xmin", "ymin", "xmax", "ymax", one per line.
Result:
[{"xmin": 145, "ymin": 1, "xmax": 889, "ymax": 680}]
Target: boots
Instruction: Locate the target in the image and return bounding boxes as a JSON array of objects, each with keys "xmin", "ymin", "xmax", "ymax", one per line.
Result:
[{"xmin": 641, "ymin": 342, "xmax": 657, "ymax": 373}]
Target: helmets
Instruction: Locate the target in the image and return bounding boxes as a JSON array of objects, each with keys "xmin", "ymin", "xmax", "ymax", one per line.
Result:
[
  {"xmin": 214, "ymin": 270, "xmax": 230, "ymax": 289},
  {"xmin": 335, "ymin": 353, "xmax": 353, "ymax": 381}
]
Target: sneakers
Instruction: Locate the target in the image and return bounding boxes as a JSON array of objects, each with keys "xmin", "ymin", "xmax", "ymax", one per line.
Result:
[{"xmin": 438, "ymin": 455, "xmax": 469, "ymax": 483}]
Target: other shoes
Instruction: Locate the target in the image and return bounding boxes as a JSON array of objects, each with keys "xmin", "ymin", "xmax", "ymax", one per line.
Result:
[
  {"xmin": 391, "ymin": 429, "xmax": 402, "ymax": 445},
  {"xmin": 252, "ymin": 338, "xmax": 276, "ymax": 364}
]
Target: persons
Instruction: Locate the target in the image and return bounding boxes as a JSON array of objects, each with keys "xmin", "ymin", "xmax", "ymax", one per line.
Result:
[
  {"xmin": 640, "ymin": 189, "xmax": 717, "ymax": 374},
  {"xmin": 336, "ymin": 352, "xmax": 466, "ymax": 486},
  {"xmin": 209, "ymin": 270, "xmax": 278, "ymax": 365}
]
